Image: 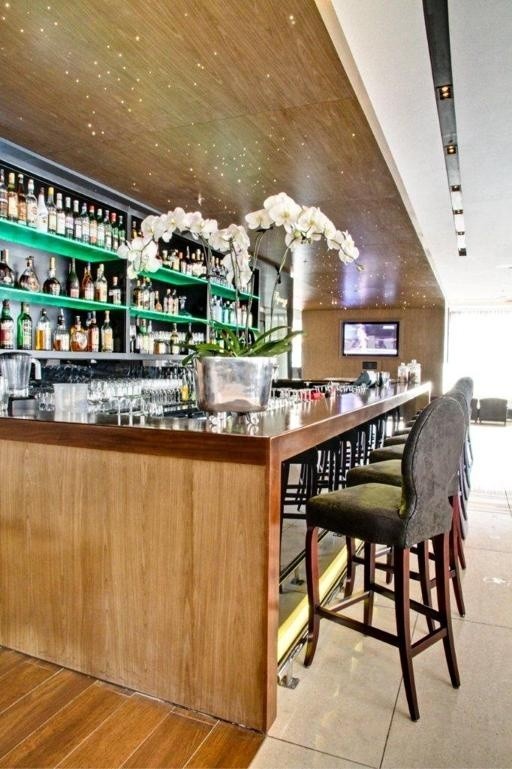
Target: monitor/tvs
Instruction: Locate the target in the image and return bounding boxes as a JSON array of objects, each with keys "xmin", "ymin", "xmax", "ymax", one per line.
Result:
[{"xmin": 343, "ymin": 321, "xmax": 399, "ymax": 356}]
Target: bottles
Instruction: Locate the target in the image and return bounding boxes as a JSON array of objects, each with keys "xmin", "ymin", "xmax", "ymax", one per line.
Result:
[
  {"xmin": 0, "ymin": 248, "xmax": 17, "ymax": 288},
  {"xmin": 170, "ymin": 322, "xmax": 180, "ymax": 354},
  {"xmin": 0, "ymin": 169, "xmax": 126, "ymax": 254},
  {"xmin": 210, "ymin": 255, "xmax": 253, "ymax": 293},
  {"xmin": 29, "ymin": 359, "xmax": 198, "ymax": 411},
  {"xmin": 18, "ymin": 255, "xmax": 41, "ymax": 294},
  {"xmin": 85, "ymin": 311, "xmax": 101, "ymax": 351},
  {"xmin": 184, "ymin": 322, "xmax": 195, "ymax": 354},
  {"xmin": 108, "ymin": 276, "xmax": 122, "ymax": 305},
  {"xmin": 100, "ymin": 310, "xmax": 114, "ymax": 352},
  {"xmin": 81, "ymin": 259, "xmax": 96, "ymax": 301},
  {"xmin": 210, "ymin": 329, "xmax": 232, "ymax": 353},
  {"xmin": 52, "ymin": 306, "xmax": 71, "ymax": 351},
  {"xmin": 133, "ymin": 314, "xmax": 154, "ymax": 355},
  {"xmin": 210, "ymin": 294, "xmax": 253, "ymax": 328},
  {"xmin": 71, "ymin": 315, "xmax": 88, "ymax": 351},
  {"xmin": 132, "ymin": 220, "xmax": 208, "ymax": 279},
  {"xmin": 407, "ymin": 359, "xmax": 421, "ymax": 384},
  {"xmin": 16, "ymin": 301, "xmax": 33, "ymax": 350},
  {"xmin": 43, "ymin": 255, "xmax": 61, "ymax": 295},
  {"xmin": 60, "ymin": 257, "xmax": 81, "ymax": 298},
  {"xmin": 134, "ymin": 275, "xmax": 193, "ymax": 316},
  {"xmin": 93, "ymin": 263, "xmax": 108, "ymax": 302},
  {"xmin": 0, "ymin": 298, "xmax": 14, "ymax": 349},
  {"xmin": 397, "ymin": 362, "xmax": 409, "ymax": 384},
  {"xmin": 35, "ymin": 307, "xmax": 51, "ymax": 350}
]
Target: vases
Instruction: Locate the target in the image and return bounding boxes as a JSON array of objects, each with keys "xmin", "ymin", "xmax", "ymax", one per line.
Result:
[{"xmin": 191, "ymin": 355, "xmax": 278, "ymax": 414}]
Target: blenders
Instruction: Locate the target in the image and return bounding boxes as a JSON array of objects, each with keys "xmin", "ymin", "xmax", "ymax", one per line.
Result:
[{"xmin": 1, "ymin": 352, "xmax": 41, "ymax": 410}]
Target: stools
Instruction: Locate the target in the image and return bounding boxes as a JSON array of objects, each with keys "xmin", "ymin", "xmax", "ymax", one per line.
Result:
[{"xmin": 280, "ymin": 405, "xmax": 399, "ymax": 598}]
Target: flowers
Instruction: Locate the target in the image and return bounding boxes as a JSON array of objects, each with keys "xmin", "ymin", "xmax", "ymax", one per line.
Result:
[{"xmin": 113, "ymin": 184, "xmax": 369, "ymax": 366}]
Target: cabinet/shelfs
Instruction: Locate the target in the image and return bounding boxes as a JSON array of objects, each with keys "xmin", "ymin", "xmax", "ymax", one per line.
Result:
[{"xmin": 1, "ymin": 156, "xmax": 263, "ymax": 364}]
